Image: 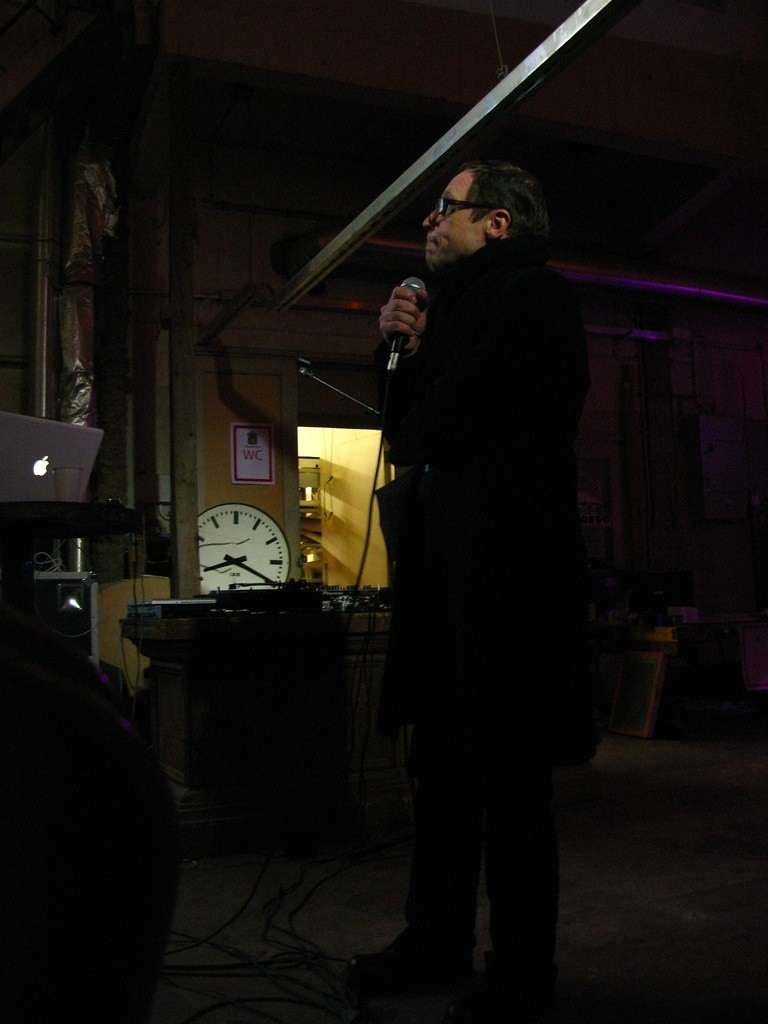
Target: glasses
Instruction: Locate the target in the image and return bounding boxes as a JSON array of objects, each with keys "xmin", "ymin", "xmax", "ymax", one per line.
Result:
[{"xmin": 435, "ymin": 196, "xmax": 498, "ymax": 214}]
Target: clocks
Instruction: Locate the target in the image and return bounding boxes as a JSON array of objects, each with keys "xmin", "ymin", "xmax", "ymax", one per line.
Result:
[{"xmin": 196, "ymin": 502, "xmax": 291, "ymax": 595}]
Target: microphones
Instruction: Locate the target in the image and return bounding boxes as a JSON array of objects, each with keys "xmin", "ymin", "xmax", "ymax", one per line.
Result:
[{"xmin": 388, "ymin": 277, "xmax": 425, "ymax": 380}]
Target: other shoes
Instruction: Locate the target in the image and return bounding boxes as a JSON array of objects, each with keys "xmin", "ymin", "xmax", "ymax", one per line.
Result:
[
  {"xmin": 446, "ymin": 963, "xmax": 556, "ymax": 1024},
  {"xmin": 348, "ymin": 926, "xmax": 474, "ymax": 997}
]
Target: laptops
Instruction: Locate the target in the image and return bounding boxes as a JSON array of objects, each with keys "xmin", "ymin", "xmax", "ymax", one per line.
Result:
[{"xmin": 0, "ymin": 410, "xmax": 105, "ymax": 502}]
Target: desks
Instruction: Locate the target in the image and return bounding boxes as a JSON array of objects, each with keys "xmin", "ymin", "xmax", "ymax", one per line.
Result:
[
  {"xmin": 119, "ymin": 611, "xmax": 415, "ymax": 861},
  {"xmin": 631, "ymin": 619, "xmax": 768, "ymax": 741}
]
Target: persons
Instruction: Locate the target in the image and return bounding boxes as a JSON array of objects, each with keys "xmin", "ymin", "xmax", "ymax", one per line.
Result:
[{"xmin": 342, "ymin": 159, "xmax": 589, "ymax": 1023}]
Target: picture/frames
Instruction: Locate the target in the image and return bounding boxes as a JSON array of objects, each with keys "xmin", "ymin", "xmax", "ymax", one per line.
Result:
[{"xmin": 607, "ymin": 651, "xmax": 667, "ymax": 737}]
